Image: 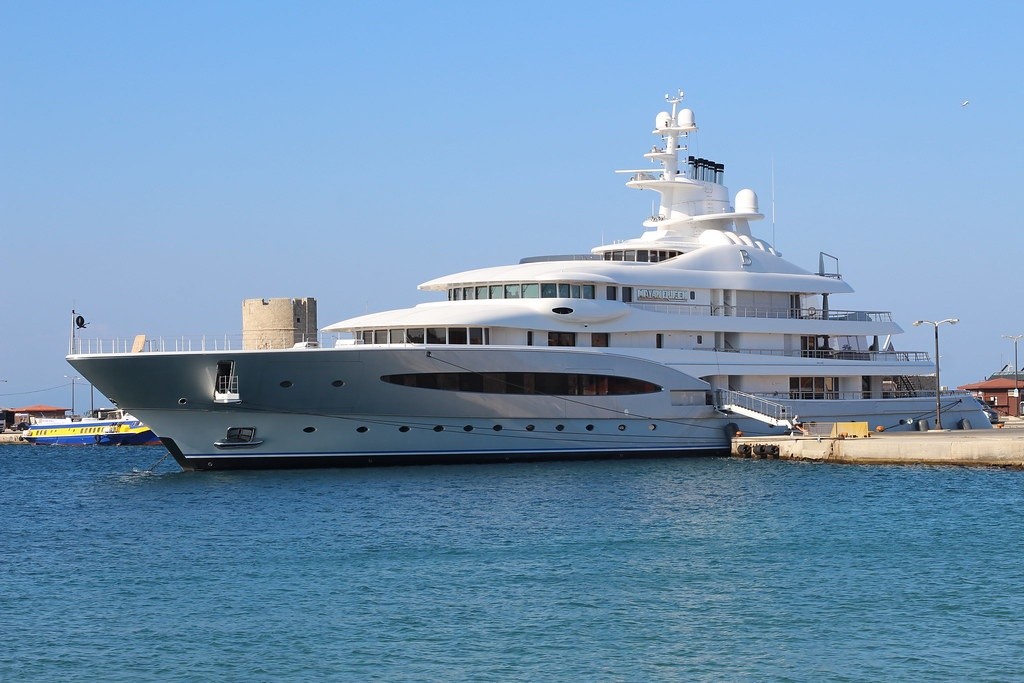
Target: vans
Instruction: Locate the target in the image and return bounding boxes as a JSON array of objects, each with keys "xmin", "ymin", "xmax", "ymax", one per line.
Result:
[{"xmin": 974, "ymin": 397, "xmax": 998, "ymax": 424}]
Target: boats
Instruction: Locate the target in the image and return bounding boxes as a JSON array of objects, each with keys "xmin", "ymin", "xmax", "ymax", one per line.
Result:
[
  {"xmin": 22, "ymin": 411, "xmax": 159, "ymax": 446},
  {"xmin": 64, "ymin": 91, "xmax": 995, "ymax": 471}
]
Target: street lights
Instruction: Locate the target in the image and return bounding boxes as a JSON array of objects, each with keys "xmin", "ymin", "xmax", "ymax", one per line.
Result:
[
  {"xmin": 1002, "ymin": 335, "xmax": 1022, "ymax": 416},
  {"xmin": 913, "ymin": 319, "xmax": 959, "ymax": 430}
]
